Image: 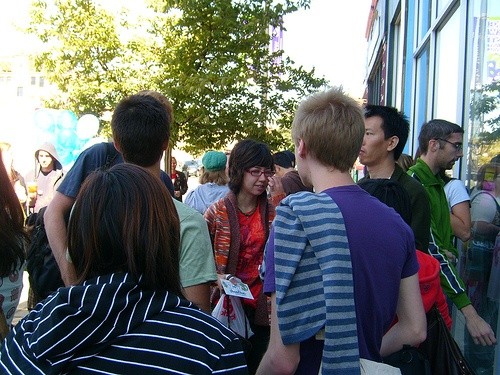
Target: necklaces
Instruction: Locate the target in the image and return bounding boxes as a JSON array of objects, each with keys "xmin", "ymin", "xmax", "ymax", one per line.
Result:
[{"xmin": 238, "ymin": 198, "xmax": 255, "ymax": 215}]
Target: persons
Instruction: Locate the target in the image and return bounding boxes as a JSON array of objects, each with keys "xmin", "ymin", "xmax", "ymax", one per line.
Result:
[
  {"xmin": 460, "ymin": 155, "xmax": 500, "ymax": 375},
  {"xmin": 186, "ymin": 139, "xmax": 315, "ymax": 375},
  {"xmin": 0, "ymin": 164, "xmax": 249, "ymax": 375},
  {"xmin": 254, "ymin": 88, "xmax": 426, "ymax": 375},
  {"xmin": 0, "ymin": 90, "xmax": 218, "ymax": 343},
  {"xmin": 357, "ymin": 104, "xmax": 497, "ymax": 358}
]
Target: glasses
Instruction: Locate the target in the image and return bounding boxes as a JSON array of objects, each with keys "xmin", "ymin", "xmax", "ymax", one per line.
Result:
[
  {"xmin": 247, "ymin": 169, "xmax": 276, "ymax": 178},
  {"xmin": 436, "ymin": 137, "xmax": 463, "ymax": 150}
]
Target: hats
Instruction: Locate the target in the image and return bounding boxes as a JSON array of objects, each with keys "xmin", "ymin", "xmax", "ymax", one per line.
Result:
[
  {"xmin": 202, "ymin": 151, "xmax": 227, "ymax": 171},
  {"xmin": 282, "ymin": 150, "xmax": 295, "ymax": 161}
]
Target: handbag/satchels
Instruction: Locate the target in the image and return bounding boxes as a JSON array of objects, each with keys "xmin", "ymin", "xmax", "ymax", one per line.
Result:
[
  {"xmin": 227, "ymin": 326, "xmax": 253, "ymax": 359},
  {"xmin": 461, "ymin": 238, "xmax": 496, "ymax": 283},
  {"xmin": 382, "ymin": 304, "xmax": 475, "ymax": 375},
  {"xmin": 27, "ymin": 207, "xmax": 80, "ymax": 302},
  {"xmin": 212, "ymin": 293, "xmax": 255, "ymax": 339}
]
